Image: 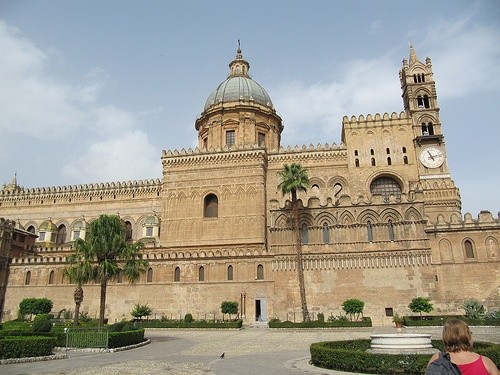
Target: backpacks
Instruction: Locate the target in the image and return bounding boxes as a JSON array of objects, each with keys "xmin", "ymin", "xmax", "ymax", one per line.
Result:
[{"xmin": 426, "ymin": 351, "xmax": 462, "ymax": 375}]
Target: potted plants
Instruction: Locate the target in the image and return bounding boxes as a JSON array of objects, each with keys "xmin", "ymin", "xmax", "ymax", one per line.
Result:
[{"xmin": 392, "ymin": 313, "xmax": 405, "ymax": 333}]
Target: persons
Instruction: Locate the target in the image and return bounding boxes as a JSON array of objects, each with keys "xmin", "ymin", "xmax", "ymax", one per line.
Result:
[{"xmin": 424, "ymin": 318, "xmax": 499, "ymax": 374}]
